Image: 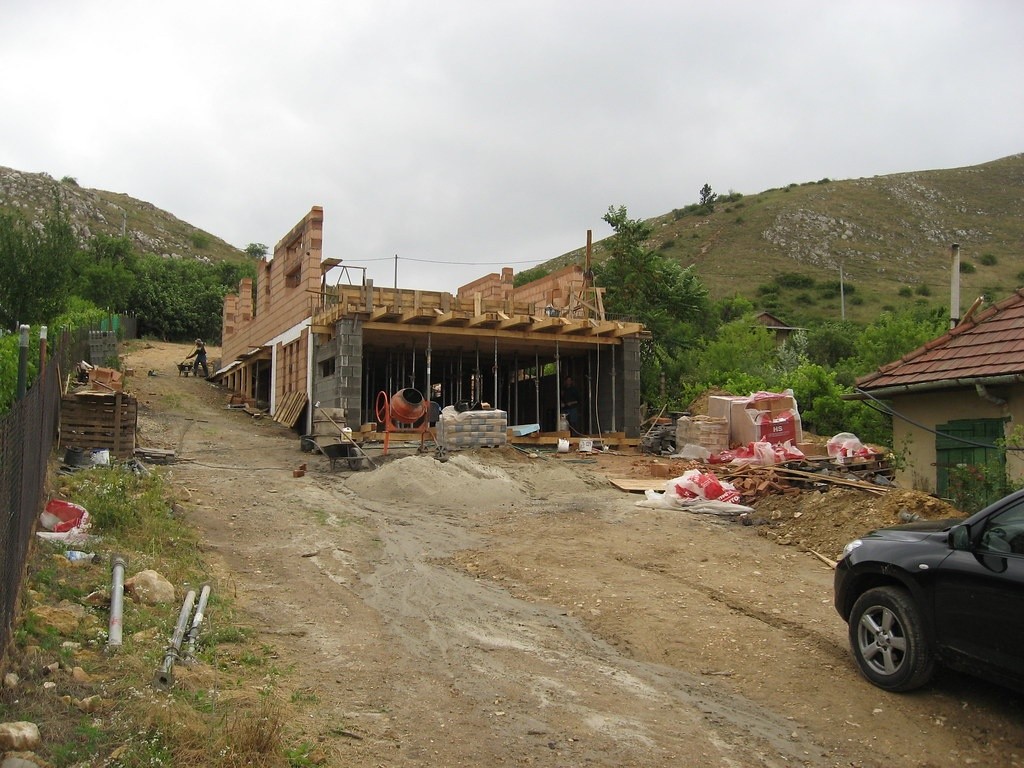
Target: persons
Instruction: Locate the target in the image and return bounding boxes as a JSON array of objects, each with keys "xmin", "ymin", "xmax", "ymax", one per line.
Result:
[{"xmin": 186, "ymin": 339, "xmax": 209, "ymax": 376}]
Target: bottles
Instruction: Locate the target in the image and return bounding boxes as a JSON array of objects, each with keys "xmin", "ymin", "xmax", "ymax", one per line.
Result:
[{"xmin": 341, "ymin": 426, "xmax": 352, "ymax": 442}]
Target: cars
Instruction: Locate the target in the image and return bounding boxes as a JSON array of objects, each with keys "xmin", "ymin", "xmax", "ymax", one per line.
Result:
[{"xmin": 832, "ymin": 488, "xmax": 1024, "ymax": 694}]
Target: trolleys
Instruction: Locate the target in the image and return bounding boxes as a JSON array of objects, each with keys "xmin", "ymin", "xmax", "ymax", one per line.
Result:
[{"xmin": 173, "ymin": 360, "xmax": 194, "ymax": 377}]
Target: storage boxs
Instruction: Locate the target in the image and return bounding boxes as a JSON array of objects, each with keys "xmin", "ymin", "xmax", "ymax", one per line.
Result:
[
  {"xmin": 88, "ymin": 366, "xmax": 124, "ymax": 393},
  {"xmin": 61, "ymin": 392, "xmax": 137, "ymax": 459}
]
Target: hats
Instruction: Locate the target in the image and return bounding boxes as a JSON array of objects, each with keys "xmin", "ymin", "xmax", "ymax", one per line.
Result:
[{"xmin": 195, "ymin": 339, "xmax": 202, "ymax": 344}]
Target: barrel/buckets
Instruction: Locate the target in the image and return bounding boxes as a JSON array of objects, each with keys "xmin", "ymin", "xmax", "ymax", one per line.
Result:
[
  {"xmin": 301, "ymin": 435, "xmax": 314, "ymax": 451},
  {"xmin": 579, "ymin": 439, "xmax": 593, "ymax": 452},
  {"xmin": 64, "ymin": 446, "xmax": 83, "ymax": 466},
  {"xmin": 557, "ymin": 439, "xmax": 569, "ymax": 453},
  {"xmin": 91, "ymin": 448, "xmax": 109, "ymax": 464}
]
